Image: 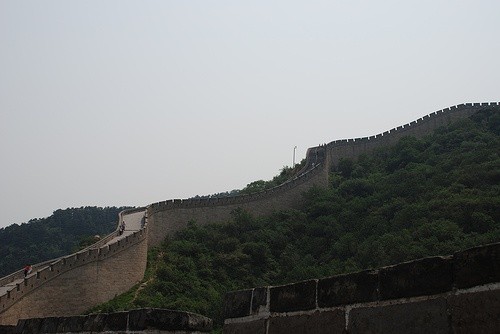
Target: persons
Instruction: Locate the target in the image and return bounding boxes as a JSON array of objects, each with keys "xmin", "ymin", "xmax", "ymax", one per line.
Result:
[
  {"xmin": 118, "ymin": 220, "xmax": 126, "ymax": 236},
  {"xmin": 23, "ymin": 264, "xmax": 33, "ymax": 278},
  {"xmin": 292, "ymin": 162, "xmax": 318, "ymax": 179}
]
